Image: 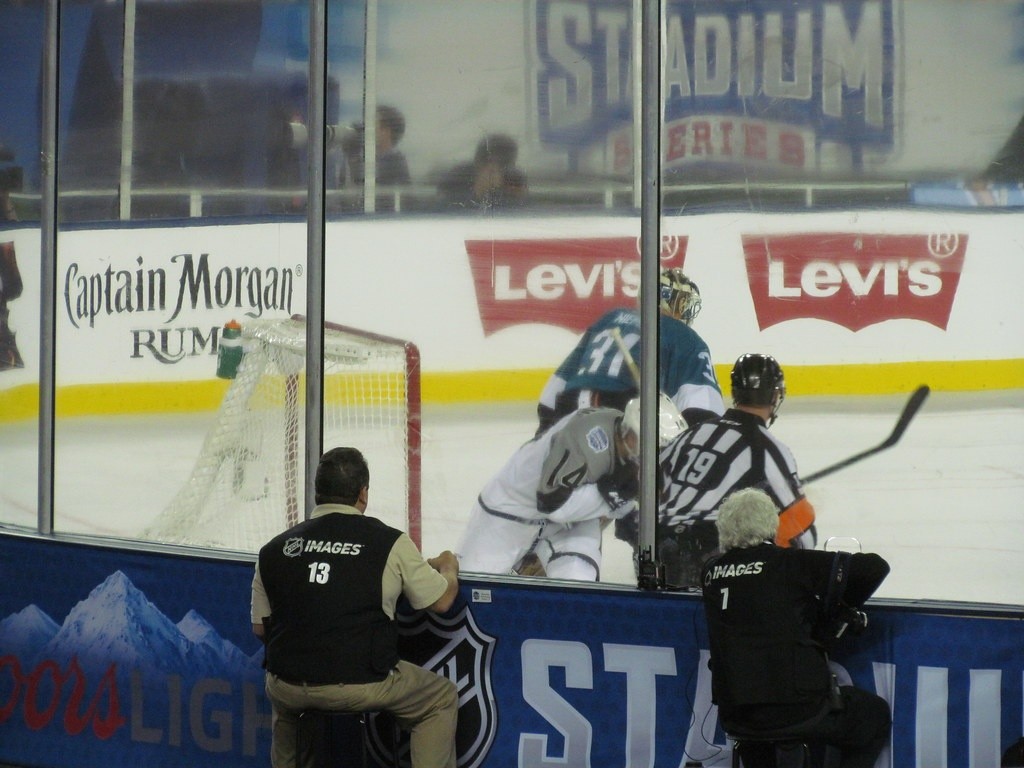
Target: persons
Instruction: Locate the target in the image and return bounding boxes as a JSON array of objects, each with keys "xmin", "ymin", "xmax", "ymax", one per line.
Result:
[
  {"xmin": 346, "ymin": 104, "xmax": 417, "ymax": 213},
  {"xmin": 633, "ymin": 355, "xmax": 817, "ymax": 591},
  {"xmin": 252, "ymin": 448, "xmax": 459, "ymax": 768},
  {"xmin": 456, "ymin": 389, "xmax": 688, "ymax": 582},
  {"xmin": 535, "ymin": 267, "xmax": 725, "ymax": 542},
  {"xmin": 700, "ymin": 488, "xmax": 893, "ymax": 768},
  {"xmin": 436, "ymin": 134, "xmax": 532, "ymax": 215}
]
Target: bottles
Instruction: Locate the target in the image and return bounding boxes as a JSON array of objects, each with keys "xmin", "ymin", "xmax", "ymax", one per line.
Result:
[{"xmin": 215, "ymin": 319, "xmax": 242, "ymax": 379}]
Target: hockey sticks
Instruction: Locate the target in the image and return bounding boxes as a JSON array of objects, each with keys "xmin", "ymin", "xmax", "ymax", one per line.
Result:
[
  {"xmin": 797, "ymin": 383, "xmax": 932, "ymax": 488},
  {"xmin": 612, "ymin": 326, "xmax": 642, "ymax": 391}
]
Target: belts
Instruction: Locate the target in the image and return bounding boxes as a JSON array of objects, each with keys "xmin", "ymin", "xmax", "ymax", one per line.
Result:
[{"xmin": 277, "ymin": 672, "xmax": 343, "ymax": 687}]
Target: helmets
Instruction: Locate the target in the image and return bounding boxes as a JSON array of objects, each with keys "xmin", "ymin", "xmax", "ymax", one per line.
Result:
[
  {"xmin": 623, "ymin": 392, "xmax": 688, "ymax": 449},
  {"xmin": 731, "ymin": 353, "xmax": 784, "ymax": 403},
  {"xmin": 659, "ymin": 268, "xmax": 701, "ymax": 325}
]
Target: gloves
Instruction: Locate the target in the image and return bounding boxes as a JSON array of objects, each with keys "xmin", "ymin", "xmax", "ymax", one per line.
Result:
[
  {"xmin": 536, "ymin": 404, "xmax": 557, "ymax": 435},
  {"xmin": 597, "ymin": 461, "xmax": 641, "ymax": 509}
]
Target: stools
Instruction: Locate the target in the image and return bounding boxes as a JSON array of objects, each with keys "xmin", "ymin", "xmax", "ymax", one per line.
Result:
[
  {"xmin": 726, "ymin": 734, "xmax": 822, "ymax": 768},
  {"xmin": 294, "ymin": 710, "xmax": 399, "ymax": 767}
]
